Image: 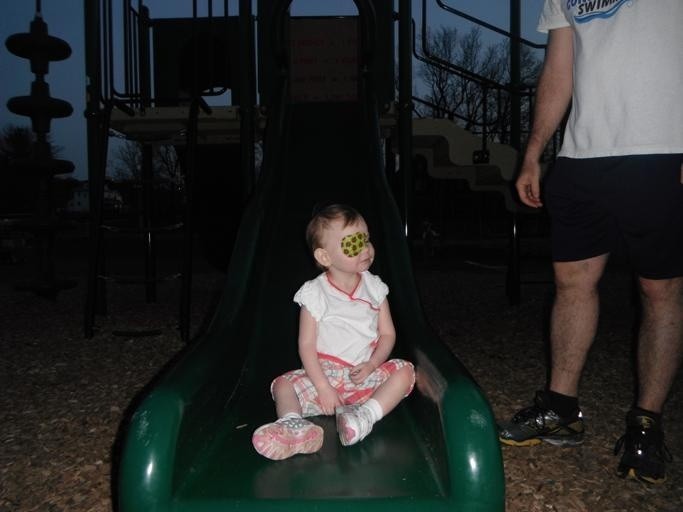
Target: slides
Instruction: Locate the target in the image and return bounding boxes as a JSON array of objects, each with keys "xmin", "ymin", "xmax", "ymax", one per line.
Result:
[{"xmin": 114, "ymin": 0, "xmax": 504, "ymax": 511}]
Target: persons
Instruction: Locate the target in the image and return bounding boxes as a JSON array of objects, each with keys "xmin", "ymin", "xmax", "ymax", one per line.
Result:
[
  {"xmin": 250, "ymin": 204, "xmax": 417, "ymax": 463},
  {"xmin": 495, "ymin": 0, "xmax": 680, "ymax": 483}
]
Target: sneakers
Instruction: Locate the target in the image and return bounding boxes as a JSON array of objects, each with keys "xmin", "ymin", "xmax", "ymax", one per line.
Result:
[
  {"xmin": 335, "ymin": 404, "xmax": 370, "ymax": 446},
  {"xmin": 614, "ymin": 408, "xmax": 672, "ymax": 483},
  {"xmin": 496, "ymin": 390, "xmax": 584, "ymax": 446},
  {"xmin": 252, "ymin": 416, "xmax": 324, "ymax": 461}
]
